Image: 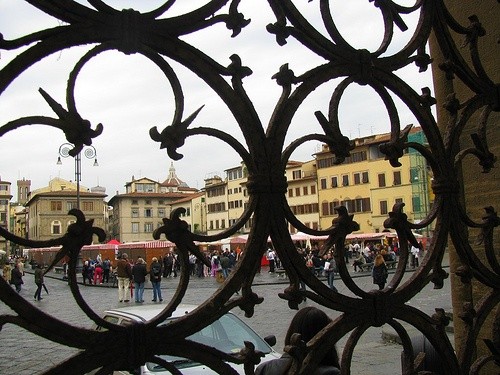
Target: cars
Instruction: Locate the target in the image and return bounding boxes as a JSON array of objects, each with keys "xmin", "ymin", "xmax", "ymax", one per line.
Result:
[{"xmin": 89, "ymin": 303, "xmax": 283, "ymax": 375}]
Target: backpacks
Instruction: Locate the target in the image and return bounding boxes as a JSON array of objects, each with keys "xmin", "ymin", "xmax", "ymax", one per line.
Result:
[{"xmin": 214, "ymin": 258, "xmax": 219, "ymax": 264}]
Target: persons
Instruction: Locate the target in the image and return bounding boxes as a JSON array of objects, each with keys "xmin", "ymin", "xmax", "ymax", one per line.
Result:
[
  {"xmin": 325, "ymin": 252, "xmax": 337, "ymax": 288},
  {"xmin": 33, "ymin": 263, "xmax": 45, "ymax": 302},
  {"xmin": 371, "ymin": 254, "xmax": 389, "ymax": 290},
  {"xmin": 10, "ymin": 264, "xmax": 25, "ymax": 292},
  {"xmin": 266, "ymin": 241, "xmax": 425, "ymax": 277},
  {"xmin": 253, "ymin": 308, "xmax": 342, "ymax": 375},
  {"xmin": 81, "ymin": 252, "xmax": 111, "ymax": 286},
  {"xmin": 149, "ymin": 257, "xmax": 163, "ymax": 302},
  {"xmin": 117, "ymin": 252, "xmax": 133, "ymax": 302},
  {"xmin": 158, "ymin": 247, "xmax": 244, "ymax": 280},
  {"xmin": 131, "ymin": 257, "xmax": 148, "ymax": 304},
  {"xmin": 11, "ymin": 254, "xmax": 30, "ymax": 275},
  {"xmin": 3, "ymin": 262, "xmax": 12, "ymax": 284}
]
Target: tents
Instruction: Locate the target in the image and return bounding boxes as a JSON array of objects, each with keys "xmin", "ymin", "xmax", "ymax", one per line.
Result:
[
  {"xmin": 207, "ymin": 232, "xmax": 396, "ymax": 260},
  {"xmin": 27, "ymin": 239, "xmax": 176, "ymax": 271}
]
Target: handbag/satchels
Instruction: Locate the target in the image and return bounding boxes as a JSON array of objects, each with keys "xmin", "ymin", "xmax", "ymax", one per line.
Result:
[{"xmin": 324, "ymin": 262, "xmax": 330, "ymax": 271}]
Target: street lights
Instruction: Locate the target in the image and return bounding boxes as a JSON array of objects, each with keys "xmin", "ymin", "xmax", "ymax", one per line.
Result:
[
  {"xmin": 194, "ymin": 202, "xmax": 207, "ymax": 232},
  {"xmin": 411, "ymin": 165, "xmax": 434, "ymax": 237},
  {"xmin": 56, "ymin": 142, "xmax": 99, "ymax": 209}
]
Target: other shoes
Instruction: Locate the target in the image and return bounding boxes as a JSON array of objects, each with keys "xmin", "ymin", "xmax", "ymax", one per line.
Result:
[
  {"xmin": 152, "ymin": 299, "xmax": 156, "ymax": 301},
  {"xmin": 135, "ymin": 301, "xmax": 140, "ymax": 303},
  {"xmin": 160, "ymin": 299, "xmax": 163, "ymax": 301},
  {"xmin": 124, "ymin": 300, "xmax": 129, "ymax": 302},
  {"xmin": 141, "ymin": 300, "xmax": 144, "ymax": 302},
  {"xmin": 120, "ymin": 300, "xmax": 122, "ymax": 302}
]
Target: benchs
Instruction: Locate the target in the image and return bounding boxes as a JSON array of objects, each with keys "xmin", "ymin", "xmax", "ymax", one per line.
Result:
[
  {"xmin": 360, "ymin": 260, "xmax": 399, "ymax": 272},
  {"xmin": 315, "ymin": 266, "xmax": 339, "ymax": 277}
]
